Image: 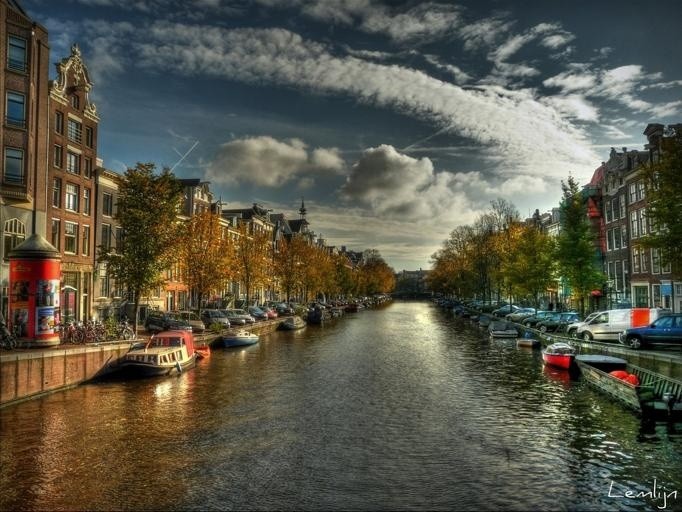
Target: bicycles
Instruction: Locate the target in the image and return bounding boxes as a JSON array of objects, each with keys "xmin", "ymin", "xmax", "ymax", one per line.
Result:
[{"xmin": 0, "ymin": 314, "xmax": 136, "ymax": 350}]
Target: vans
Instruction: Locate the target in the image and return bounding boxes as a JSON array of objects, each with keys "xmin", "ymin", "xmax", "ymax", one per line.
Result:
[{"xmin": 170, "ymin": 311, "xmax": 205, "ymax": 332}]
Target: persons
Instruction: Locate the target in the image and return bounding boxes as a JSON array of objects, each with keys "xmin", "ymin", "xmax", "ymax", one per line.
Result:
[
  {"xmin": 13, "ymin": 280, "xmax": 27, "ymax": 302},
  {"xmin": 15, "ymin": 309, "xmax": 26, "ymax": 337}
]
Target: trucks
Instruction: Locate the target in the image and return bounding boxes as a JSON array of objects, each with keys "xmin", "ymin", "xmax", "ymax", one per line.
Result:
[{"xmin": 577, "ymin": 308, "xmax": 671, "ymax": 342}]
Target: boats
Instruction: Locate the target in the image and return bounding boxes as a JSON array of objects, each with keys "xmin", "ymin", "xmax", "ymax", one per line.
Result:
[
  {"xmin": 541, "ymin": 365, "xmax": 577, "ymax": 386},
  {"xmin": 515, "ymin": 337, "xmax": 539, "ymax": 347},
  {"xmin": 122, "ymin": 327, "xmax": 213, "ymax": 377},
  {"xmin": 221, "ymin": 330, "xmax": 259, "ymax": 348},
  {"xmin": 306, "ymin": 295, "xmax": 392, "ymax": 323},
  {"xmin": 542, "ymin": 342, "xmax": 575, "ymax": 368},
  {"xmin": 432, "ymin": 298, "xmax": 521, "ymax": 339},
  {"xmin": 575, "ymin": 352, "xmax": 682, "ymax": 424},
  {"xmin": 278, "ymin": 315, "xmax": 307, "ymax": 330}
]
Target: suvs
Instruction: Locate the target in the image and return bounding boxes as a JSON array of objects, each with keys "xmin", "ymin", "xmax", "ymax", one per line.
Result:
[
  {"xmin": 145, "ymin": 309, "xmax": 192, "ymax": 334},
  {"xmin": 568, "ymin": 312, "xmax": 599, "ymax": 339},
  {"xmin": 618, "ymin": 312, "xmax": 682, "ymax": 349}
]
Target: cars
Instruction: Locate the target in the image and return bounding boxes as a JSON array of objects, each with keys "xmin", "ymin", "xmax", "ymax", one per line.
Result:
[
  {"xmin": 461, "ymin": 299, "xmax": 578, "ymax": 333},
  {"xmin": 223, "ymin": 293, "xmax": 391, "ymax": 326},
  {"xmin": 201, "ymin": 309, "xmax": 231, "ymax": 329}
]
